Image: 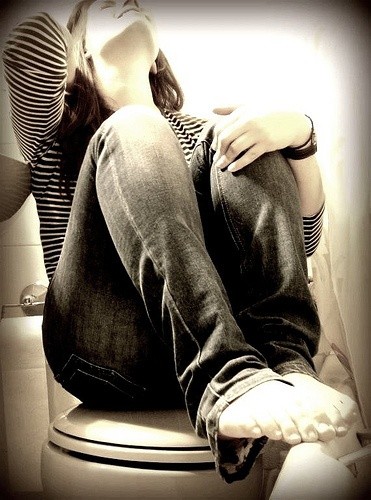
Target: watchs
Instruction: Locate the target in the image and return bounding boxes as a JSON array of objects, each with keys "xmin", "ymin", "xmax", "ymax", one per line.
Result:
[{"xmin": 280, "ymin": 134, "xmax": 317, "ymax": 160}]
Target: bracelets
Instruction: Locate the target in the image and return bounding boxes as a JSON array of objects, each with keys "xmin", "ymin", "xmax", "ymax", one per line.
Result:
[{"xmin": 290, "ymin": 114, "xmax": 314, "ymax": 149}]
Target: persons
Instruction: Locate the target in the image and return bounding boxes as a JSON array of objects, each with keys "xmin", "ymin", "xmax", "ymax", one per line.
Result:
[{"xmin": 0, "ymin": 0, "xmax": 371, "ymax": 500}]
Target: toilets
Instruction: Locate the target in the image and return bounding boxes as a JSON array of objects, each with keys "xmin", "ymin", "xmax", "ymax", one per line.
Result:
[{"xmin": 40, "ymin": 404, "xmax": 265, "ymax": 500}]
